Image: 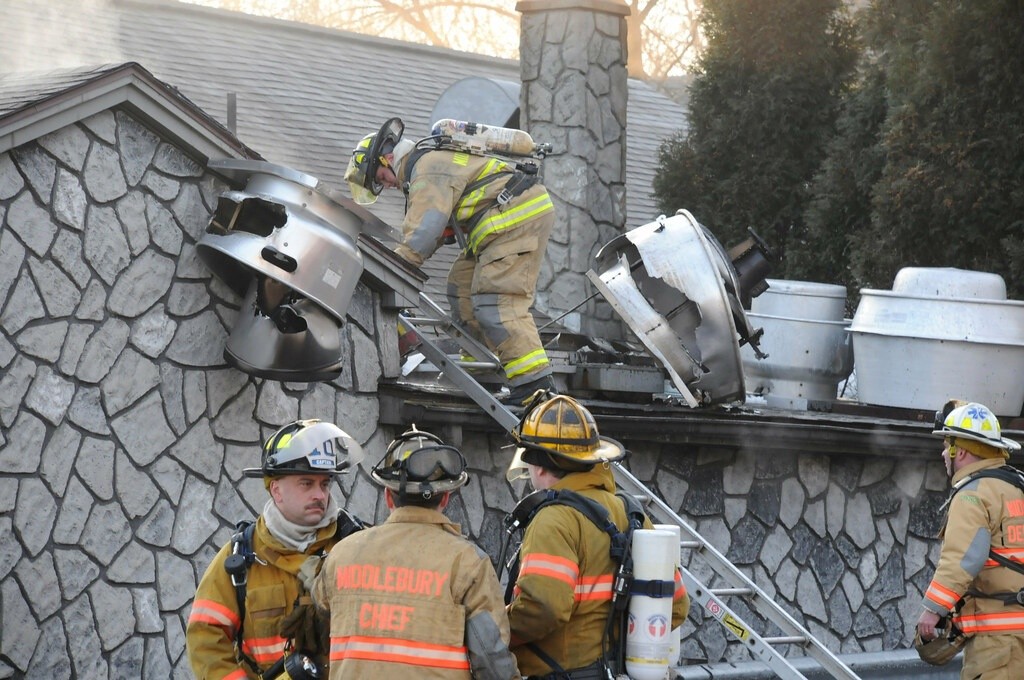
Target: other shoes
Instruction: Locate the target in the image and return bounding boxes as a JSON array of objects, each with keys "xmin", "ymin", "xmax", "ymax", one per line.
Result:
[{"xmin": 498, "ymin": 371, "xmax": 555, "ymax": 405}]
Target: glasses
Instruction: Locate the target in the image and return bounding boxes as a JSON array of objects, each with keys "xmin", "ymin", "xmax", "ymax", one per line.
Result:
[
  {"xmin": 934, "ymin": 410, "xmax": 946, "ymax": 430},
  {"xmin": 400, "ymin": 444, "xmax": 466, "ymax": 479}
]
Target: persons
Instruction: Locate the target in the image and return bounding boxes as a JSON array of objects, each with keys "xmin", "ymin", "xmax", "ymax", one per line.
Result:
[
  {"xmin": 916, "ymin": 400, "xmax": 1024, "ymax": 680},
  {"xmin": 343, "ymin": 117, "xmax": 558, "ymax": 402},
  {"xmin": 501, "ymin": 387, "xmax": 692, "ymax": 679},
  {"xmin": 185, "ymin": 417, "xmax": 376, "ymax": 680},
  {"xmin": 310, "ymin": 423, "xmax": 524, "ymax": 680}
]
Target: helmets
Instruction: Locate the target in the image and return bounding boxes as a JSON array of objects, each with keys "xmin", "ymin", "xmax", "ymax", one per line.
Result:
[
  {"xmin": 932, "ymin": 400, "xmax": 1022, "ymax": 449},
  {"xmin": 371, "ymin": 428, "xmax": 469, "ymax": 495},
  {"xmin": 343, "ymin": 116, "xmax": 405, "ymax": 203},
  {"xmin": 518, "ymin": 393, "xmax": 624, "ymax": 462},
  {"xmin": 242, "ymin": 419, "xmax": 363, "ymax": 474}
]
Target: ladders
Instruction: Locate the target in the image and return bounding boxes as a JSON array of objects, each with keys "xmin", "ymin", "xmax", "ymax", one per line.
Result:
[{"xmin": 398, "ymin": 291, "xmax": 863, "ymax": 680}]
